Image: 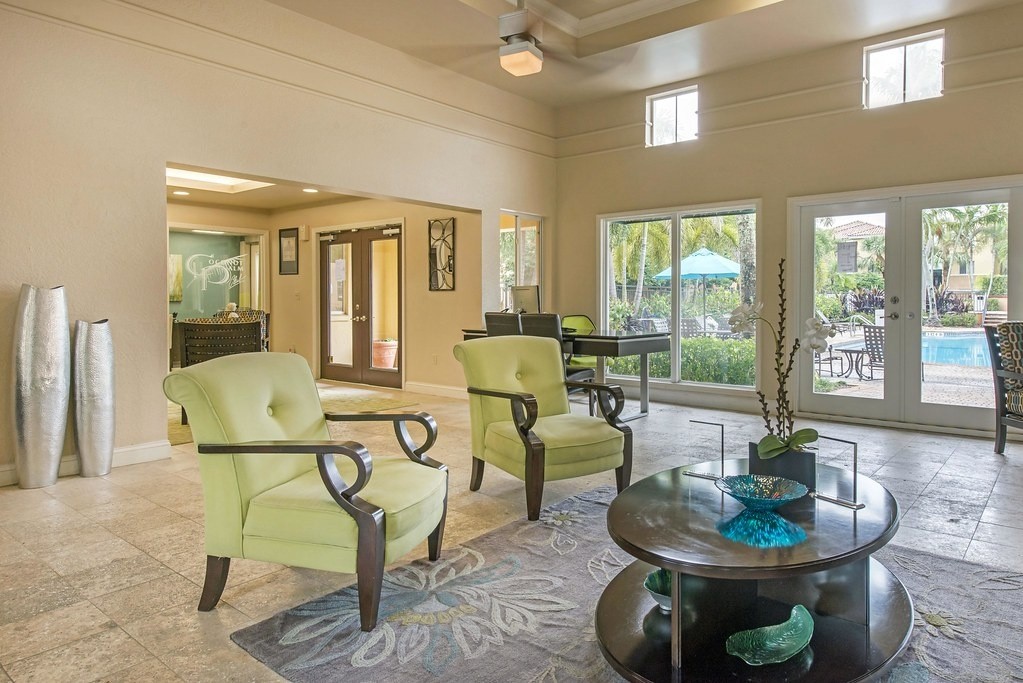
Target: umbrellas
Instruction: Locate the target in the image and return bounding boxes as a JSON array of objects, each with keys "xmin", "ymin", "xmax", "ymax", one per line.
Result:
[{"xmin": 652, "ymin": 246, "xmax": 740, "ymax": 338}]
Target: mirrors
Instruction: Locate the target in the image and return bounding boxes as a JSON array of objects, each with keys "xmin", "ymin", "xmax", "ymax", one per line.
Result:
[{"xmin": 428, "ymin": 217, "xmax": 454, "ymax": 291}]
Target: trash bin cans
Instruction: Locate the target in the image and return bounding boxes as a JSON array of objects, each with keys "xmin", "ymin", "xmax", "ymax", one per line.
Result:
[{"xmin": 974, "ymin": 294, "xmax": 986, "ymax": 311}]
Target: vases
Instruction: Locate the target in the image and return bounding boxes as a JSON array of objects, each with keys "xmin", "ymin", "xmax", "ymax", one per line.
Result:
[{"xmin": 748, "ymin": 442, "xmax": 816, "ymax": 492}]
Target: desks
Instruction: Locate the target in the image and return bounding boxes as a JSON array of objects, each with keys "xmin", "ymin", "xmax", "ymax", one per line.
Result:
[
  {"xmin": 593, "ymin": 455, "xmax": 915, "ymax": 683},
  {"xmin": 835, "ymin": 347, "xmax": 871, "ymax": 381},
  {"xmin": 462, "ymin": 329, "xmax": 671, "ymax": 423}
]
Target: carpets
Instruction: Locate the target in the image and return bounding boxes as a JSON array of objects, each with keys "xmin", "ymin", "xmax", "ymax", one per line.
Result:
[
  {"xmin": 168, "ymin": 419, "xmax": 193, "ymax": 446},
  {"xmin": 320, "ymin": 394, "xmax": 420, "ymax": 413},
  {"xmin": 230, "ymin": 488, "xmax": 1023, "ymax": 683}
]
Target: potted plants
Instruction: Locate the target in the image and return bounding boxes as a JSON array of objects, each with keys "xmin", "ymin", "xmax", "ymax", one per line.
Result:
[{"xmin": 374, "ymin": 338, "xmax": 398, "ymax": 368}]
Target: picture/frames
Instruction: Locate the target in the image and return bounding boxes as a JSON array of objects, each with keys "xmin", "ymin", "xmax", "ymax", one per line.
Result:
[
  {"xmin": 448, "ymin": 255, "xmax": 452, "ymax": 272},
  {"xmin": 279, "ymin": 227, "xmax": 299, "ymax": 275}
]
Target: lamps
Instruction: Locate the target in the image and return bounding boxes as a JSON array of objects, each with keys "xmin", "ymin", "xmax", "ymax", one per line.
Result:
[{"xmin": 497, "ymin": 0, "xmax": 544, "ymax": 78}]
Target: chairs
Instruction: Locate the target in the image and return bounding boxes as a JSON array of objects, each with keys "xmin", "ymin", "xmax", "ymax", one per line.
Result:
[
  {"xmin": 864, "ymin": 324, "xmax": 925, "ymax": 382},
  {"xmin": 560, "ymin": 314, "xmax": 614, "ymax": 370},
  {"xmin": 162, "ymin": 351, "xmax": 448, "ymax": 632},
  {"xmin": 178, "ymin": 317, "xmax": 263, "ymax": 424},
  {"xmin": 453, "ymin": 335, "xmax": 633, "ymax": 521},
  {"xmin": 213, "ymin": 307, "xmax": 271, "ymax": 351},
  {"xmin": 984, "ymin": 320, "xmax": 1023, "ymax": 453},
  {"xmin": 486, "ymin": 311, "xmax": 595, "ymax": 417},
  {"xmin": 652, "ymin": 313, "xmax": 738, "ymax": 338},
  {"xmin": 816, "ymin": 309, "xmax": 847, "ymax": 335},
  {"xmin": 814, "ymin": 344, "xmax": 843, "ymax": 378}
]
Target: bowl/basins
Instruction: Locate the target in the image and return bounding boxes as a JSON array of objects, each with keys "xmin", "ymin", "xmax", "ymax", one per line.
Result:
[{"xmin": 644, "ymin": 569, "xmax": 672, "ymax": 611}]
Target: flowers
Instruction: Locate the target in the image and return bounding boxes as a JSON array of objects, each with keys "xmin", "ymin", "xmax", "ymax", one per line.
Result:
[{"xmin": 729, "ymin": 258, "xmax": 836, "ymax": 459}]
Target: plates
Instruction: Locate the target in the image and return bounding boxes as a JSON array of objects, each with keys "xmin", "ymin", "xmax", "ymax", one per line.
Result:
[
  {"xmin": 715, "ymin": 473, "xmax": 809, "ymax": 511},
  {"xmin": 725, "ymin": 604, "xmax": 815, "ymax": 666}
]
